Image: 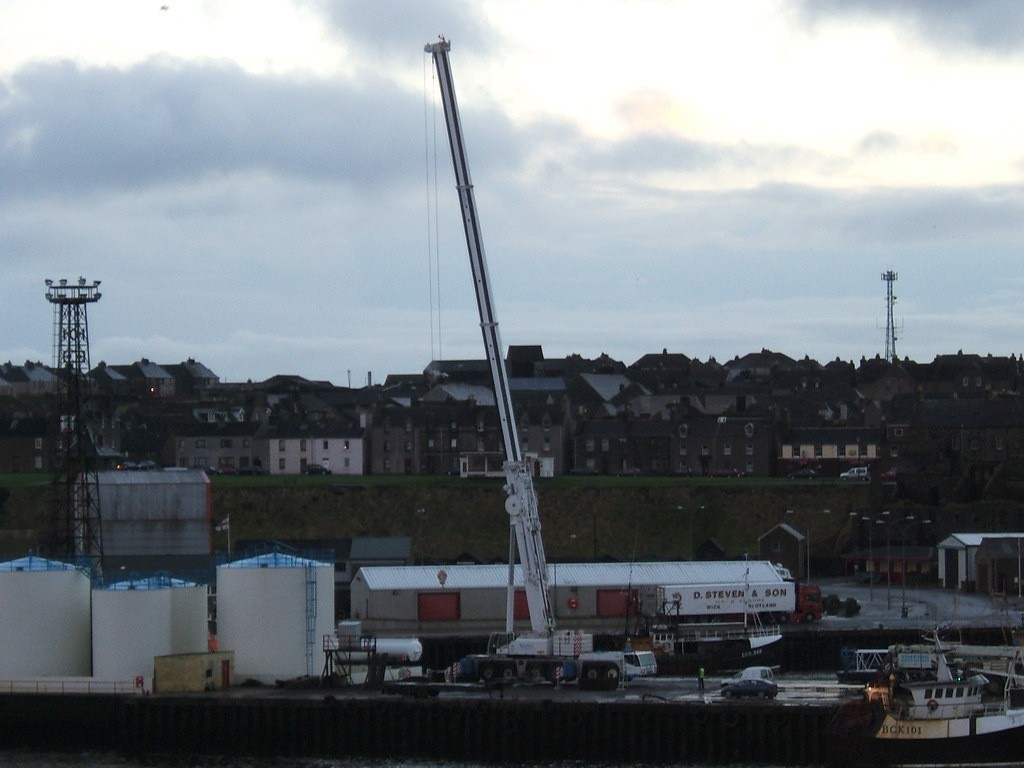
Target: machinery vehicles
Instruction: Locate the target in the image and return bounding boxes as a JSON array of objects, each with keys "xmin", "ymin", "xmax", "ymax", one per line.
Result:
[{"xmin": 424, "ymin": 32, "xmax": 663, "ymax": 695}]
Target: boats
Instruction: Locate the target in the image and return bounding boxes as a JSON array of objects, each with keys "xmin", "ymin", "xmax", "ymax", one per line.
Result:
[{"xmin": 868, "ymin": 618, "xmax": 1024, "ymax": 768}]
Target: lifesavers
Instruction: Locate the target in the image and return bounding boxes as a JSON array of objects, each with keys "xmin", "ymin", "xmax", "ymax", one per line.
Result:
[
  {"xmin": 927, "ymin": 699, "xmax": 939, "ymax": 711},
  {"xmin": 208, "ymin": 632, "xmax": 218, "ymax": 652}
]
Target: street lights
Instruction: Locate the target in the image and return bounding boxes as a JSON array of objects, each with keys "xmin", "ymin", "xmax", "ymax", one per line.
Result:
[
  {"xmin": 674, "ymin": 505, "xmax": 706, "ymax": 562},
  {"xmin": 824, "ymin": 509, "xmax": 931, "ymax": 617},
  {"xmin": 786, "ymin": 509, "xmax": 832, "ymax": 582}
]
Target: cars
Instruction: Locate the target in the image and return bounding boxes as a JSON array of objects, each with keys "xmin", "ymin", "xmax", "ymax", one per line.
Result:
[
  {"xmin": 706, "ymin": 467, "xmax": 745, "ymax": 479},
  {"xmin": 839, "ymin": 466, "xmax": 871, "ymax": 481},
  {"xmin": 720, "ymin": 679, "xmax": 779, "ymax": 700},
  {"xmin": 787, "ymin": 468, "xmax": 817, "ymax": 480},
  {"xmin": 721, "ymin": 667, "xmax": 774, "ymax": 688},
  {"xmin": 135, "ymin": 460, "xmax": 157, "ymax": 471},
  {"xmin": 674, "ymin": 466, "xmax": 694, "ymax": 477},
  {"xmin": 301, "ymin": 464, "xmax": 331, "ymax": 475},
  {"xmin": 445, "ymin": 466, "xmax": 460, "ymax": 476},
  {"xmin": 570, "ymin": 466, "xmax": 599, "ymax": 477}
]
